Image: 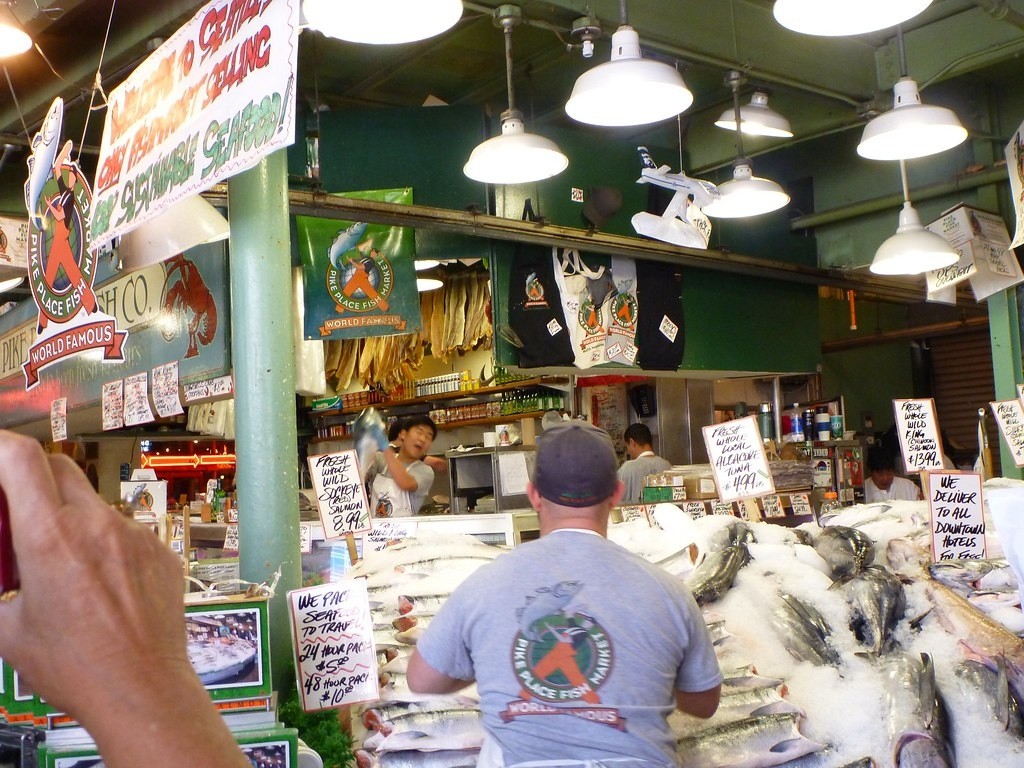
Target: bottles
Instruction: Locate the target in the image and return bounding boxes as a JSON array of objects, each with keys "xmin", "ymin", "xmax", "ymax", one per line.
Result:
[
  {"xmin": 317, "ymin": 359, "xmax": 564, "ymax": 438},
  {"xmin": 757, "ymin": 403, "xmax": 773, "ymax": 442},
  {"xmin": 790, "ymin": 403, "xmax": 804, "ymax": 442},
  {"xmin": 820, "ymin": 492, "xmax": 842, "ymax": 516},
  {"xmin": 801, "ymin": 412, "xmax": 814, "ymax": 440},
  {"xmin": 735, "ymin": 401, "xmax": 748, "ymax": 419},
  {"xmin": 214, "ymin": 478, "xmax": 225, "ymax": 512}
]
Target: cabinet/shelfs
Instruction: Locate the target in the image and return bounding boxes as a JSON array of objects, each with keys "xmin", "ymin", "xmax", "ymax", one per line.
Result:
[{"xmin": 306, "ymin": 371, "xmax": 579, "ymax": 466}]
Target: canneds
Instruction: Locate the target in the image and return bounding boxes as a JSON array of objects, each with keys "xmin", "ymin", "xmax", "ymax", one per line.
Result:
[{"xmin": 427, "ymin": 401, "xmax": 502, "ymax": 425}]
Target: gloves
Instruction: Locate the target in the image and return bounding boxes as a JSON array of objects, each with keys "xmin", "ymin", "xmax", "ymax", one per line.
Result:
[{"xmin": 370, "ymin": 425, "xmax": 389, "ymax": 451}]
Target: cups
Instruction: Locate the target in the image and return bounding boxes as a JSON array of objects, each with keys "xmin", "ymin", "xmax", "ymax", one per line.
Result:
[
  {"xmin": 816, "ymin": 413, "xmax": 830, "ymax": 441},
  {"xmin": 829, "ymin": 416, "xmax": 843, "ymax": 441}
]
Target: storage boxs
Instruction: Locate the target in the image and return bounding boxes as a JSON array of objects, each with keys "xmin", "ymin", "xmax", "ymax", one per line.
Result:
[{"xmin": 682, "ymin": 474, "xmax": 718, "ymax": 499}]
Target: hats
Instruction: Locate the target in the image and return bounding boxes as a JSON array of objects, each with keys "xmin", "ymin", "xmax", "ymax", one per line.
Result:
[{"xmin": 533, "ymin": 420, "xmax": 618, "ymax": 506}]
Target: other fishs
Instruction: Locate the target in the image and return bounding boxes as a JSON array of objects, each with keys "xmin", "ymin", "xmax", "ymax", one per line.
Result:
[
  {"xmin": 853, "ymin": 637, "xmax": 958, "ymax": 768},
  {"xmin": 726, "ymin": 523, "xmax": 758, "ymax": 546},
  {"xmin": 775, "ymin": 589, "xmax": 819, "ymax": 628},
  {"xmin": 872, "ymin": 565, "xmax": 905, "ymax": 632},
  {"xmin": 953, "ymin": 660, "xmax": 1024, "ymax": 742},
  {"xmin": 769, "ymin": 595, "xmax": 847, "ymax": 682},
  {"xmin": 826, "ymin": 525, "xmax": 877, "ymax": 591},
  {"xmin": 817, "ymin": 612, "xmax": 832, "ymax": 631},
  {"xmin": 925, "ymin": 577, "xmax": 1024, "ymax": 705},
  {"xmin": 675, "ymin": 544, "xmax": 749, "ymax": 602},
  {"xmin": 632, "ymin": 545, "xmax": 830, "ymax": 767},
  {"xmin": 819, "ymin": 503, "xmax": 933, "ymax": 583},
  {"xmin": 928, "ymin": 557, "xmax": 1019, "ymax": 597},
  {"xmin": 801, "ymin": 599, "xmax": 831, "ymax": 638},
  {"xmin": 842, "ymin": 567, "xmax": 896, "ymax": 660},
  {"xmin": 350, "ymin": 530, "xmax": 510, "ymax": 768},
  {"xmin": 784, "ymin": 529, "xmax": 814, "ymax": 547},
  {"xmin": 814, "ymin": 525, "xmax": 860, "ymax": 578},
  {"xmin": 985, "ymin": 602, "xmax": 1024, "ymax": 634},
  {"xmin": 966, "ymin": 588, "xmax": 1021, "ymax": 612}
]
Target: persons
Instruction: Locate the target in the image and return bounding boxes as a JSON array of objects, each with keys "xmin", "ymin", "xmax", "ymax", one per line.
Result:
[
  {"xmin": 356, "ymin": 415, "xmax": 447, "ymax": 517},
  {"xmin": 404, "ymin": 419, "xmax": 723, "ymax": 768},
  {"xmin": 865, "ymin": 449, "xmax": 922, "ymax": 501},
  {"xmin": 0, "ymin": 426, "xmax": 253, "ymax": 768},
  {"xmin": 617, "ymin": 422, "xmax": 671, "ymax": 507}
]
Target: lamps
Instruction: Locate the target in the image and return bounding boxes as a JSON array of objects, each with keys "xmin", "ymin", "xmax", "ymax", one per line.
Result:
[
  {"xmin": 701, "ymin": 68, "xmax": 791, "ymax": 218},
  {"xmin": 856, "ymin": 23, "xmax": 968, "ymax": 161},
  {"xmin": 714, "ymin": 83, "xmax": 794, "ymax": 140},
  {"xmin": 869, "ymin": 158, "xmax": 960, "ymax": 276},
  {"xmin": 120, "ymin": 192, "xmax": 232, "ymax": 273},
  {"xmin": 414, "ymin": 6, "xmax": 570, "ymax": 292},
  {"xmin": 0, "ymin": 0, "xmax": 33, "ymax": 58},
  {"xmin": 566, "ymin": 1, "xmax": 693, "ymax": 129},
  {"xmin": 773, "ymin": 0, "xmax": 934, "ymax": 39}
]
